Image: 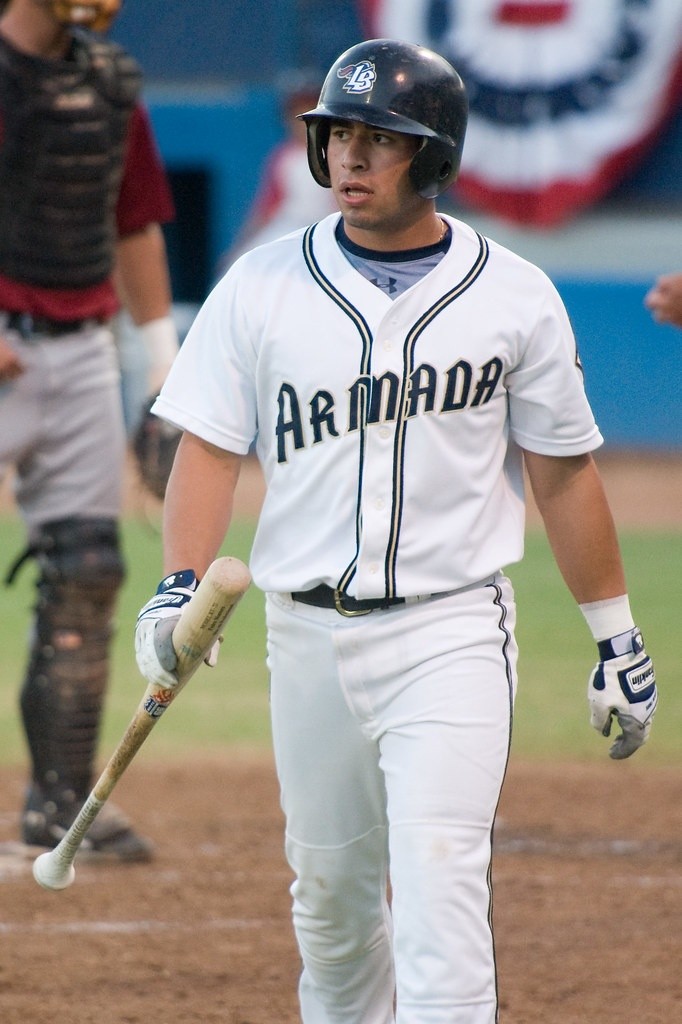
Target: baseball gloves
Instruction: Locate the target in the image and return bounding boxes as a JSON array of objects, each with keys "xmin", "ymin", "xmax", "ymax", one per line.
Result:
[{"xmin": 133, "ymin": 382, "xmax": 184, "ymax": 501}]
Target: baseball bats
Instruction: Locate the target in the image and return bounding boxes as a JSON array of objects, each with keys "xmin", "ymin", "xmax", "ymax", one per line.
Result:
[{"xmin": 35, "ymin": 554, "xmax": 253, "ymax": 891}]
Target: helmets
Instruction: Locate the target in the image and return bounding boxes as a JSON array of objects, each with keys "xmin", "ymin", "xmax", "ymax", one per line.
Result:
[{"xmin": 293, "ymin": 35, "xmax": 471, "ymax": 200}]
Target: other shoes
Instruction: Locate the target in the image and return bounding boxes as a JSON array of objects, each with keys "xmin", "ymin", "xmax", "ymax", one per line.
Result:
[{"xmin": 21, "ymin": 783, "xmax": 150, "ymax": 861}]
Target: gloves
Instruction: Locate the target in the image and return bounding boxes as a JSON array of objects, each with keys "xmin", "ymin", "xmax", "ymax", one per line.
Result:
[
  {"xmin": 586, "ymin": 624, "xmax": 659, "ymax": 761},
  {"xmin": 130, "ymin": 388, "xmax": 184, "ymax": 500},
  {"xmin": 132, "ymin": 568, "xmax": 224, "ymax": 690}
]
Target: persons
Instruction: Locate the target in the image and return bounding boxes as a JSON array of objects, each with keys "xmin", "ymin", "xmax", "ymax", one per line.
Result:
[
  {"xmin": 129, "ymin": 37, "xmax": 659, "ymax": 1023},
  {"xmin": 0, "ymin": 0, "xmax": 181, "ymax": 864}
]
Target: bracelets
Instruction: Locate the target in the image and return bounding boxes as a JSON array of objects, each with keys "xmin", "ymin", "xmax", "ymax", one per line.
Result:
[{"xmin": 136, "ymin": 316, "xmax": 179, "ymax": 371}]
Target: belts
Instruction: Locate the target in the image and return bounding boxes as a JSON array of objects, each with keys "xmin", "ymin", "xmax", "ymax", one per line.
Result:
[
  {"xmin": 293, "ymin": 583, "xmax": 442, "ymax": 618},
  {"xmin": 0, "ymin": 304, "xmax": 104, "ymax": 342}
]
